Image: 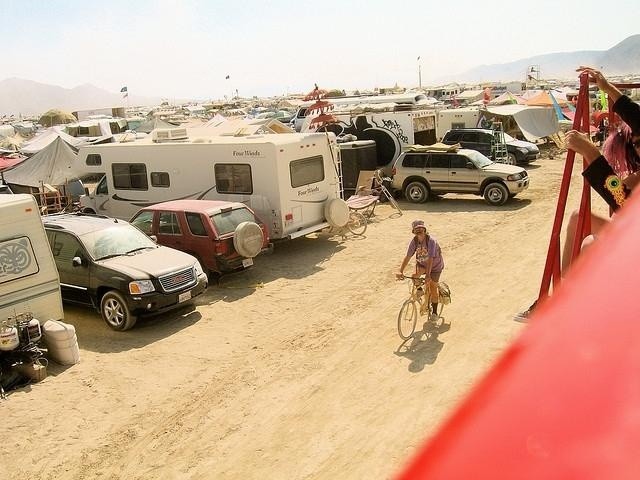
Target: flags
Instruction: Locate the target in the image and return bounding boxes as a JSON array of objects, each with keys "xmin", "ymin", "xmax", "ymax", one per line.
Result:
[{"xmin": 120, "ymin": 86, "xmax": 127, "ymax": 92}]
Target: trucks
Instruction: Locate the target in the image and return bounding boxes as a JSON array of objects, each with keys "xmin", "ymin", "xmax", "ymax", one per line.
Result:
[
  {"xmin": 0, "ymin": 193, "xmax": 67, "ymax": 340},
  {"xmin": 75, "ymin": 129, "xmax": 354, "ymax": 250},
  {"xmin": 282, "ymin": 85, "xmax": 488, "ymax": 181}
]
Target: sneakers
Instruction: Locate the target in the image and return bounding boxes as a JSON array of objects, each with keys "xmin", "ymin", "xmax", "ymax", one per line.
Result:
[
  {"xmin": 431, "ymin": 313, "xmax": 438, "ymax": 324},
  {"xmin": 514, "ymin": 301, "xmax": 537, "ymax": 323}
]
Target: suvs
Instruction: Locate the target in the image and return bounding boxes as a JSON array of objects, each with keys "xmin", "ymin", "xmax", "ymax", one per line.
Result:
[
  {"xmin": 41, "ymin": 212, "xmax": 211, "ymax": 333},
  {"xmin": 389, "ymin": 144, "xmax": 530, "ymax": 206},
  {"xmin": 443, "ymin": 128, "xmax": 541, "ymax": 167},
  {"xmin": 126, "ymin": 198, "xmax": 272, "ymax": 289}
]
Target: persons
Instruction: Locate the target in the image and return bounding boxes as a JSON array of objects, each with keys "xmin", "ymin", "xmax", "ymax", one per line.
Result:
[
  {"xmin": 602, "ymin": 123, "xmax": 639, "ymax": 192},
  {"xmin": 512, "ymin": 65, "xmax": 640, "ymax": 322},
  {"xmin": 395, "ymin": 219, "xmax": 445, "ymax": 321}
]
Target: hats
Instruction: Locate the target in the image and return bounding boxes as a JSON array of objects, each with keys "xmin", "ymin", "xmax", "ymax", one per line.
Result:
[{"xmin": 411, "ymin": 219, "xmax": 426, "ymax": 233}]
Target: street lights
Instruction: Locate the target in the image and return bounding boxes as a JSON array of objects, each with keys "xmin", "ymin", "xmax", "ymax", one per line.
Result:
[{"xmin": 415, "ymin": 56, "xmax": 425, "ymax": 88}]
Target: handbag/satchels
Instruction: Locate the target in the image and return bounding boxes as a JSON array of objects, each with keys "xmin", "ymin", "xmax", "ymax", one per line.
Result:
[{"xmin": 437, "ymin": 282, "xmax": 451, "ymax": 304}]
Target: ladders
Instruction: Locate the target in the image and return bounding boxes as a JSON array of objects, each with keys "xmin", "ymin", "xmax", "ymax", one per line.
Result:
[{"xmin": 490, "ymin": 122, "xmax": 510, "ymax": 164}]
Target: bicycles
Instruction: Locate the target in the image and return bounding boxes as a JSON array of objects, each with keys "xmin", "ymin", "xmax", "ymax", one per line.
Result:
[{"xmin": 393, "ymin": 271, "xmax": 447, "ymax": 342}]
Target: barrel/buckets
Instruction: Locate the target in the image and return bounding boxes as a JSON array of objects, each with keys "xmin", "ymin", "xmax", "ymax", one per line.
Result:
[
  {"xmin": 28, "ymin": 318, "xmax": 42, "ymax": 342},
  {"xmin": 1, "ymin": 327, "xmax": 19, "ymax": 351}
]
